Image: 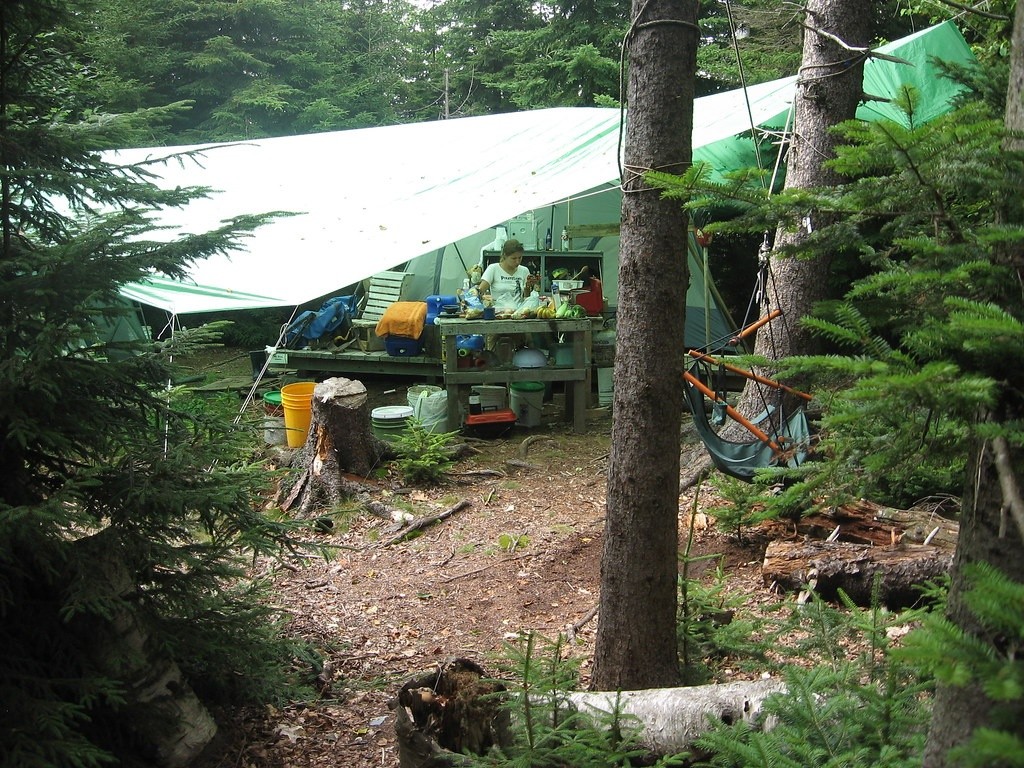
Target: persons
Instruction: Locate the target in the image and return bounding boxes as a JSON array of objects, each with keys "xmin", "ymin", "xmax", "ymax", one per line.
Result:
[{"xmin": 478, "ymin": 240, "xmax": 537, "ymax": 354}]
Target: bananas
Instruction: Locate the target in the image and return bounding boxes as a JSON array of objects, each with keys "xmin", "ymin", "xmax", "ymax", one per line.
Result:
[{"xmin": 536, "ymin": 305, "xmax": 556, "ymax": 319}]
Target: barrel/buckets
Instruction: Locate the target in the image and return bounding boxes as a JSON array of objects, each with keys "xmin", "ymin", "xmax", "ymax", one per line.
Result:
[
  {"xmin": 422, "ymin": 398, "xmax": 448, "ymax": 433},
  {"xmin": 426, "ymin": 295, "xmax": 457, "ymax": 323},
  {"xmin": 142, "ymin": 326, "xmax": 152, "ymax": 339},
  {"xmin": 263, "ymin": 391, "xmax": 288, "ymax": 446},
  {"xmin": 385, "ymin": 335, "xmax": 423, "ymax": 356},
  {"xmin": 407, "ymin": 386, "xmax": 442, "ymax": 412},
  {"xmin": 281, "ymin": 382, "xmax": 319, "ymax": 448},
  {"xmin": 510, "ymin": 381, "xmax": 546, "ymax": 427},
  {"xmin": 372, "ymin": 406, "xmax": 413, "ymax": 442},
  {"xmin": 456, "ymin": 335, "xmax": 484, "ymax": 352},
  {"xmin": 472, "ymin": 386, "xmax": 506, "ymax": 410}
]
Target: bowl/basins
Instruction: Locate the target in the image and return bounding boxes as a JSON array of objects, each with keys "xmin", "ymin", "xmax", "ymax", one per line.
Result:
[
  {"xmin": 513, "ymin": 348, "xmax": 547, "ymax": 367},
  {"xmin": 443, "ymin": 305, "xmax": 459, "ymax": 314}
]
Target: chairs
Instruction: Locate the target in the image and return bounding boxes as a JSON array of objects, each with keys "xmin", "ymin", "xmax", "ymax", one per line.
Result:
[{"xmin": 351, "ymin": 271, "xmax": 415, "ymax": 351}]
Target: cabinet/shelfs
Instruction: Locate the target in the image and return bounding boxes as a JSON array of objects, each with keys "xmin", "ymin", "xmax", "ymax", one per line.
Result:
[
  {"xmin": 482, "ymin": 249, "xmax": 617, "ymax": 313},
  {"xmin": 440, "ymin": 316, "xmax": 591, "ymax": 435},
  {"xmin": 592, "ymin": 343, "xmax": 616, "ymax": 368}
]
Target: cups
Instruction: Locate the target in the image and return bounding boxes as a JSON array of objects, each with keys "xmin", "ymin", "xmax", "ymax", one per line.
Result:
[
  {"xmin": 484, "ymin": 308, "xmax": 495, "ymax": 319},
  {"xmin": 537, "ymin": 238, "xmax": 545, "ymax": 250}
]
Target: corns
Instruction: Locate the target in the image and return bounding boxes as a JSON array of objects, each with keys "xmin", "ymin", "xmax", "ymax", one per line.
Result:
[{"xmin": 556, "ymin": 301, "xmax": 571, "ymax": 319}]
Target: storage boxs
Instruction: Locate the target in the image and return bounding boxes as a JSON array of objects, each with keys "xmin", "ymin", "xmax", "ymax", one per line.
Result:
[
  {"xmin": 508, "ymin": 220, "xmax": 537, "ymax": 251},
  {"xmin": 375, "ymin": 301, "xmax": 427, "ymax": 357}
]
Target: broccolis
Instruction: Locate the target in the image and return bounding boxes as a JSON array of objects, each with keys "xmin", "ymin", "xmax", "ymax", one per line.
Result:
[{"xmin": 571, "ymin": 305, "xmax": 587, "ymax": 318}]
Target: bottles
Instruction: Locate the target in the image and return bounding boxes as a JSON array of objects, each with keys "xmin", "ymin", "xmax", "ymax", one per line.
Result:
[
  {"xmin": 546, "ymin": 229, "xmax": 551, "ymax": 250},
  {"xmin": 469, "ymin": 390, "xmax": 482, "ymax": 415},
  {"xmin": 560, "ymin": 226, "xmax": 569, "ymax": 251},
  {"xmin": 531, "ymin": 270, "xmax": 540, "ymax": 292},
  {"xmin": 545, "ymin": 271, "xmax": 549, "ymax": 292}
]
